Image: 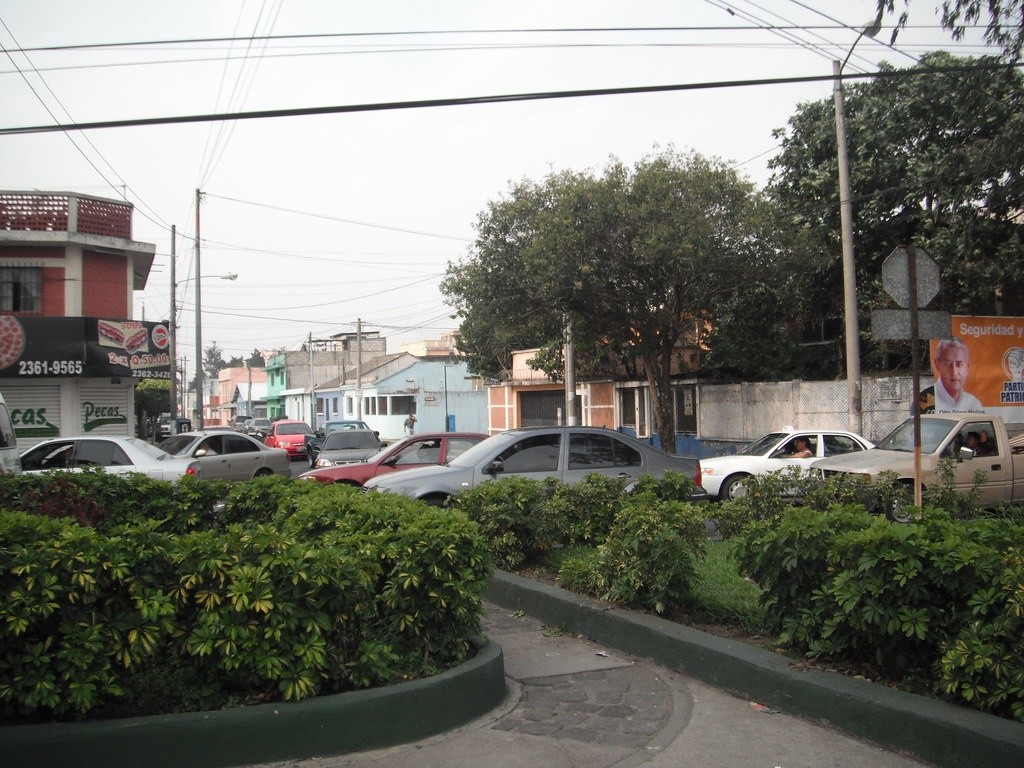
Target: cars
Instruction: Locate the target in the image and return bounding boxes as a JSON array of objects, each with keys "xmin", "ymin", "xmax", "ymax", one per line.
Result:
[
  {"xmin": 227, "ymin": 415, "xmax": 271, "ymax": 443},
  {"xmin": 154, "ymin": 430, "xmax": 293, "ymax": 484},
  {"xmin": 19, "ymin": 435, "xmax": 203, "ymax": 487},
  {"xmin": 699, "ymin": 427, "xmax": 876, "ymax": 504},
  {"xmin": 315, "ymin": 430, "xmax": 388, "ymax": 470},
  {"xmin": 299, "ymin": 431, "xmax": 518, "ymax": 493},
  {"xmin": 265, "ymin": 421, "xmax": 313, "ymax": 458},
  {"xmin": 365, "ymin": 425, "xmax": 708, "ymax": 522}
]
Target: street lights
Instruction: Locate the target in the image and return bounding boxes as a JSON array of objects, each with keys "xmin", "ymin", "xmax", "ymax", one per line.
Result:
[
  {"xmin": 169, "ymin": 273, "xmax": 239, "ymax": 434},
  {"xmin": 833, "ymin": 19, "xmax": 883, "ymax": 440}
]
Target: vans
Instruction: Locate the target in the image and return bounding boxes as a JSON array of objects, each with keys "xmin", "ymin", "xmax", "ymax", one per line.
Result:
[{"xmin": 0, "ymin": 392, "xmax": 23, "ymax": 477}]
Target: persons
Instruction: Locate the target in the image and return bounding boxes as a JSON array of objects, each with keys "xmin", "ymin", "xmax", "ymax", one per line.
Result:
[
  {"xmin": 783, "ymin": 439, "xmax": 812, "ymax": 459},
  {"xmin": 200, "ymin": 440, "xmax": 217, "ymax": 456},
  {"xmin": 947, "ymin": 429, "xmax": 990, "ymax": 458},
  {"xmin": 397, "ymin": 443, "xmax": 432, "ymax": 463},
  {"xmin": 934, "ymin": 337, "xmax": 982, "ymax": 411},
  {"xmin": 404, "ymin": 414, "xmax": 418, "ymax": 436},
  {"xmin": 374, "ymin": 431, "xmax": 382, "ymax": 442}
]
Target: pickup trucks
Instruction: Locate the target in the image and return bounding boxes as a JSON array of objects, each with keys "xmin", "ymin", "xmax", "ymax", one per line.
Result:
[
  {"xmin": 807, "ymin": 413, "xmax": 1021, "ymax": 526},
  {"xmin": 304, "ymin": 419, "xmax": 384, "ymax": 468}
]
